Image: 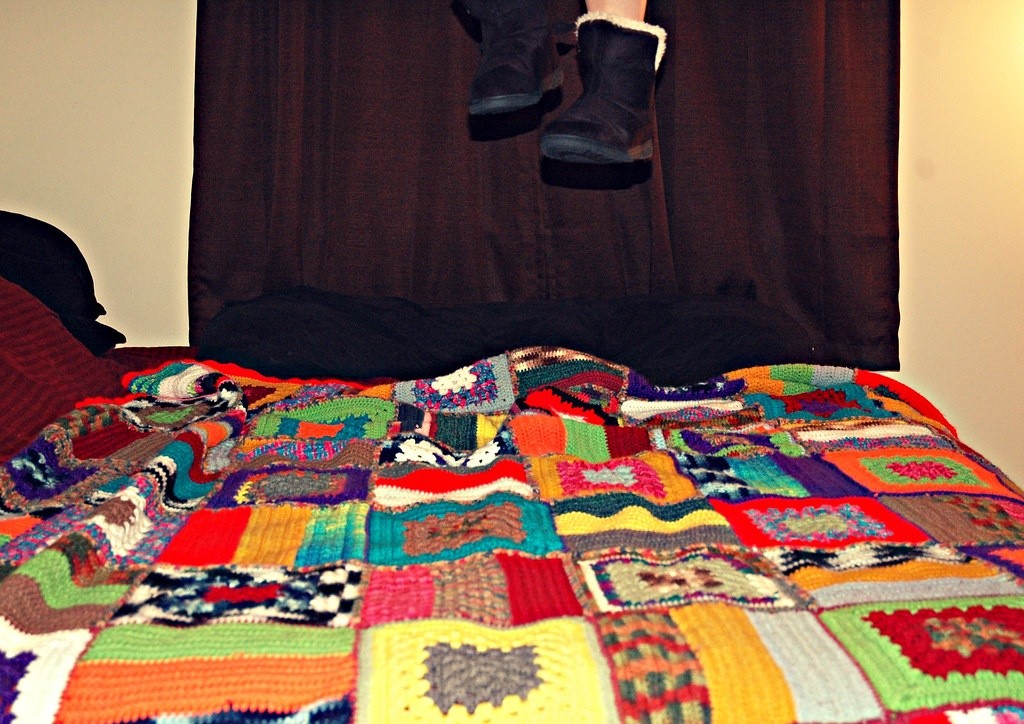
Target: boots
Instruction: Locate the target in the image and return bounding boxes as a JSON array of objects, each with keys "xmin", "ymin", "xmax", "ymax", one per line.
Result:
[
  {"xmin": 538, "ymin": 11, "xmax": 667, "ymax": 165},
  {"xmin": 462, "ymin": 0, "xmax": 564, "ymax": 116}
]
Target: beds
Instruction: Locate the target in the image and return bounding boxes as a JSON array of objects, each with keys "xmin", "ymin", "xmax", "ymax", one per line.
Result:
[{"xmin": 0, "ymin": 208, "xmax": 1024, "ymax": 723}]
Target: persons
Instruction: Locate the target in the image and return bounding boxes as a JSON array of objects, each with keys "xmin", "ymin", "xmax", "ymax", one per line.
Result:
[{"xmin": 468, "ymin": 0, "xmax": 667, "ymax": 164}]
